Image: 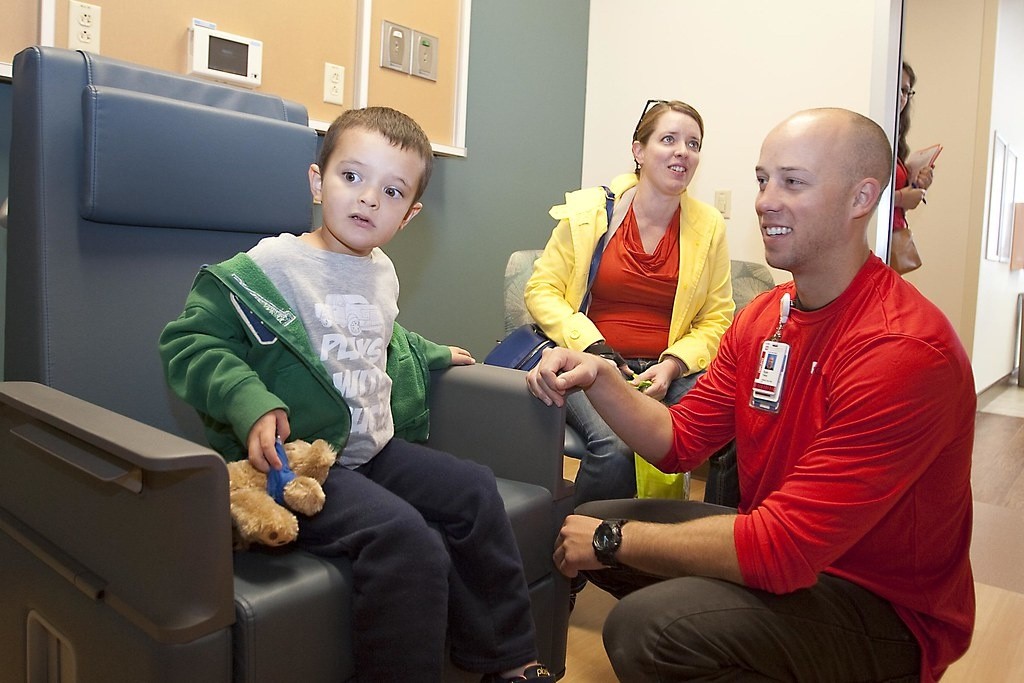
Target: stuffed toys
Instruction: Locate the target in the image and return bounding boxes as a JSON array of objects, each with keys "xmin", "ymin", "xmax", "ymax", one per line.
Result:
[{"xmin": 227, "ymin": 432, "xmax": 337, "ymax": 553}]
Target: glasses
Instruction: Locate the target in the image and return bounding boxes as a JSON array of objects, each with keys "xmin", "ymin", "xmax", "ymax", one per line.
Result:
[
  {"xmin": 901, "ymin": 87, "xmax": 915, "ymax": 98},
  {"xmin": 632, "ymin": 97, "xmax": 670, "ymax": 138}
]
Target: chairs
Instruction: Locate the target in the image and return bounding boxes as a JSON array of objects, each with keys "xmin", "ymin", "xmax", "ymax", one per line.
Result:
[
  {"xmin": 503, "ymin": 246, "xmax": 775, "ymax": 505},
  {"xmin": 0, "ymin": 46, "xmax": 581, "ymax": 682}
]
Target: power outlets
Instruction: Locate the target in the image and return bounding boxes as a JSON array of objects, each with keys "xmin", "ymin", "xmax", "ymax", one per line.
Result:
[
  {"xmin": 380, "ymin": 21, "xmax": 439, "ymax": 82},
  {"xmin": 324, "ymin": 62, "xmax": 345, "ymax": 105},
  {"xmin": 67, "ymin": 0, "xmax": 102, "ymax": 54}
]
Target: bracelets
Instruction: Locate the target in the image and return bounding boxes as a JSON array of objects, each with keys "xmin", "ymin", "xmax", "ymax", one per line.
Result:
[{"xmin": 897, "ymin": 188, "xmax": 903, "ymax": 207}]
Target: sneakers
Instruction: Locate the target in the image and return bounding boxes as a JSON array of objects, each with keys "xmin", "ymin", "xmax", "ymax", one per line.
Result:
[{"xmin": 494, "ymin": 665, "xmax": 556, "ymax": 683}]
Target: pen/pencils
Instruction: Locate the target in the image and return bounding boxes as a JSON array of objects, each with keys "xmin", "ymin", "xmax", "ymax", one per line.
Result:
[{"xmin": 911, "ymin": 182, "xmax": 927, "ymax": 205}]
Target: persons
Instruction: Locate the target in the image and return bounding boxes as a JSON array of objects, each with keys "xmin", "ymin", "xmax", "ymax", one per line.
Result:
[
  {"xmin": 524, "ymin": 99, "xmax": 735, "ymax": 613},
  {"xmin": 525, "ymin": 108, "xmax": 976, "ymax": 682},
  {"xmin": 890, "ymin": 61, "xmax": 934, "ymax": 277},
  {"xmin": 157, "ymin": 107, "xmax": 557, "ymax": 683}
]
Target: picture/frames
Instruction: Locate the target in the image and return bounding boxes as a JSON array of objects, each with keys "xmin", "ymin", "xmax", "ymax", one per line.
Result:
[{"xmin": 986, "ymin": 130, "xmax": 1018, "ymax": 267}]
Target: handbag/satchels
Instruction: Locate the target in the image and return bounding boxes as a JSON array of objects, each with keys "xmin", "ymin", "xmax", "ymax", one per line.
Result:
[
  {"xmin": 483, "ymin": 324, "xmax": 558, "ymax": 372},
  {"xmin": 891, "ymin": 228, "xmax": 922, "ymax": 275}
]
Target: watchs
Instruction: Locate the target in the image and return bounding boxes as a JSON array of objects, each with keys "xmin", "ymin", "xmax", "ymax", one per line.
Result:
[{"xmin": 593, "ymin": 518, "xmax": 630, "ymax": 569}]
